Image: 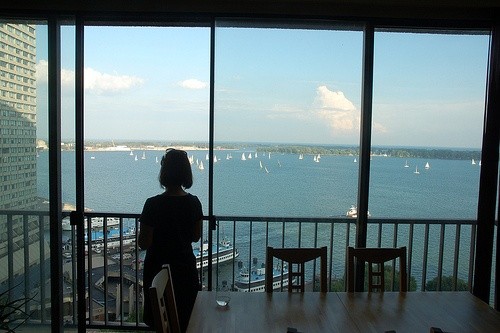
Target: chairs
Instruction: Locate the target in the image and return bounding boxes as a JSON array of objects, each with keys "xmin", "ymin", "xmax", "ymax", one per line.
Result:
[
  {"xmin": 148, "ymin": 264, "xmax": 181, "ymax": 333},
  {"xmin": 347, "ymin": 246, "xmax": 408, "ymax": 292},
  {"xmin": 265, "ymin": 246, "xmax": 327, "ymax": 292}
]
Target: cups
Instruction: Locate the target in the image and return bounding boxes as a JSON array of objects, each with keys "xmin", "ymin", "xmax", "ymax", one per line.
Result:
[{"xmin": 216, "ymin": 289, "xmax": 230, "ymax": 307}]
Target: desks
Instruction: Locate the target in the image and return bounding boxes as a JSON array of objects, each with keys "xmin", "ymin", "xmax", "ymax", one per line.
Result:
[{"xmin": 185, "ymin": 291, "xmax": 500, "ymax": 333}]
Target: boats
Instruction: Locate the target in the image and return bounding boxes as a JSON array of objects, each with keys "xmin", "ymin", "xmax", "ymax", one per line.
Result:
[
  {"xmin": 346, "ymin": 204, "xmax": 372, "ymax": 218},
  {"xmin": 68, "ymin": 219, "xmax": 142, "ymax": 255},
  {"xmin": 191, "ymin": 228, "xmax": 240, "ymax": 272},
  {"xmin": 234, "ymin": 257, "xmax": 297, "ymax": 292},
  {"xmin": 62, "ymin": 217, "xmax": 120, "ymax": 231}
]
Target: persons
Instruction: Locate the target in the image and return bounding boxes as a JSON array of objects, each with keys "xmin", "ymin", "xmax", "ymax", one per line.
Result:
[{"xmin": 138, "ymin": 148, "xmax": 203, "ymax": 333}]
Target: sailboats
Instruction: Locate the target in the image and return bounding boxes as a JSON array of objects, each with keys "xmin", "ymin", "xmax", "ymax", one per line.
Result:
[{"xmin": 90, "ymin": 149, "xmax": 482, "ymax": 175}]
